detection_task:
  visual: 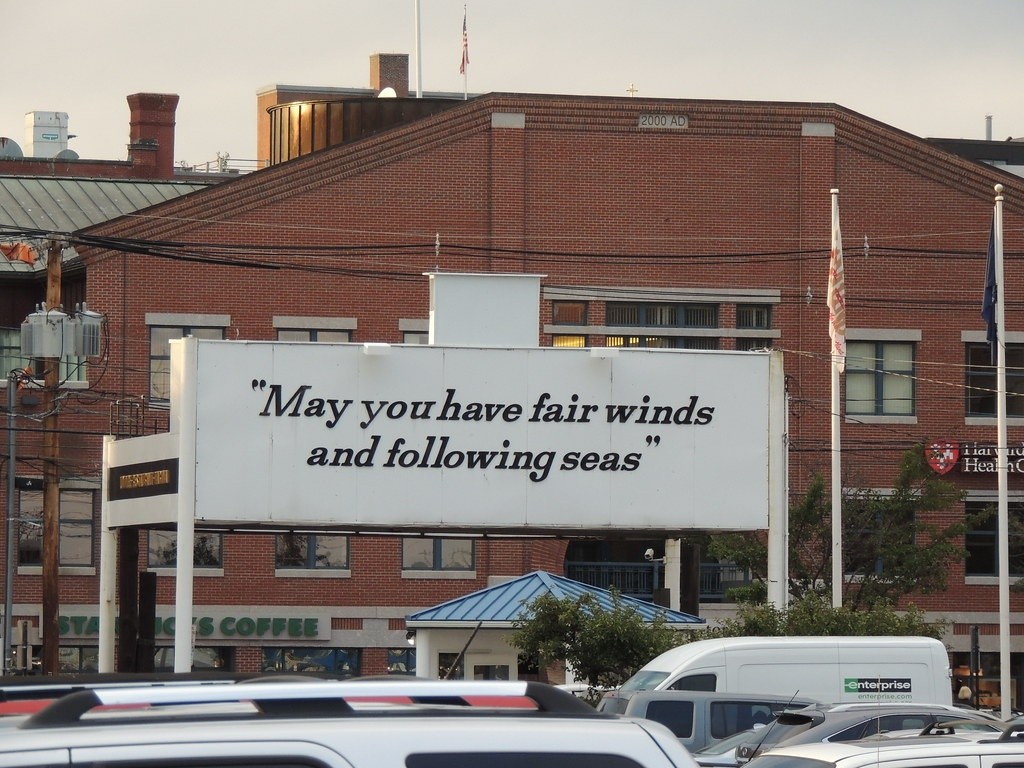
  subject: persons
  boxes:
[957,686,975,708]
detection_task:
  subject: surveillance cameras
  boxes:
[644,547,654,559]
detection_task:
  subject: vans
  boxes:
[594,636,954,752]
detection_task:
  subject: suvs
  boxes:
[689,700,1024,766]
[0,681,699,768]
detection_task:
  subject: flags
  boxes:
[459,16,470,75]
[826,206,847,374]
[980,214,998,345]
